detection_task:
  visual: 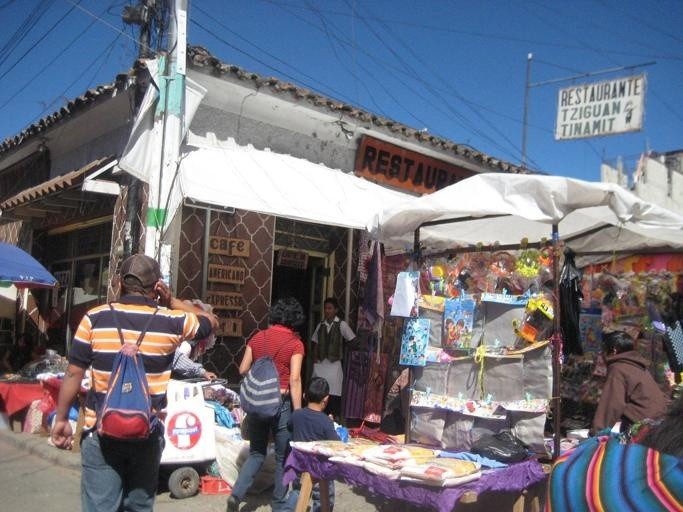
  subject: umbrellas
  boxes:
[0,242,59,289]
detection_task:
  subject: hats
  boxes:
[120,253,164,287]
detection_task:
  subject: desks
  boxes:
[0,371,94,454]
[283,438,550,512]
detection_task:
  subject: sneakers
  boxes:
[226,495,241,512]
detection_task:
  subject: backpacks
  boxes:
[240,330,299,420]
[96,303,160,442]
